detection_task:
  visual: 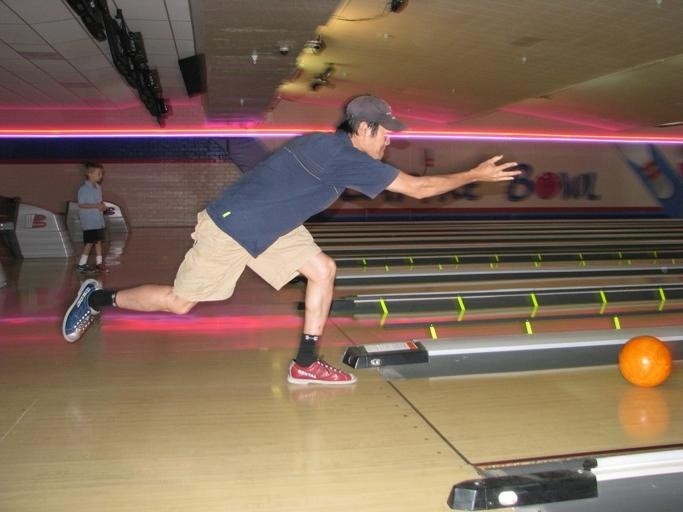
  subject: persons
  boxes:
[62,95,521,384]
[76,160,110,268]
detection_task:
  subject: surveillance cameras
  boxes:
[280,48,289,55]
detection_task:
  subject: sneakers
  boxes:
[75,263,111,276]
[61,278,104,345]
[286,353,359,387]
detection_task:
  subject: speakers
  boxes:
[178,54,202,96]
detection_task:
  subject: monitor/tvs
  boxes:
[138,62,162,126]
[67,0,105,41]
[104,11,138,89]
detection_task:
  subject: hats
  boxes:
[346,93,407,134]
